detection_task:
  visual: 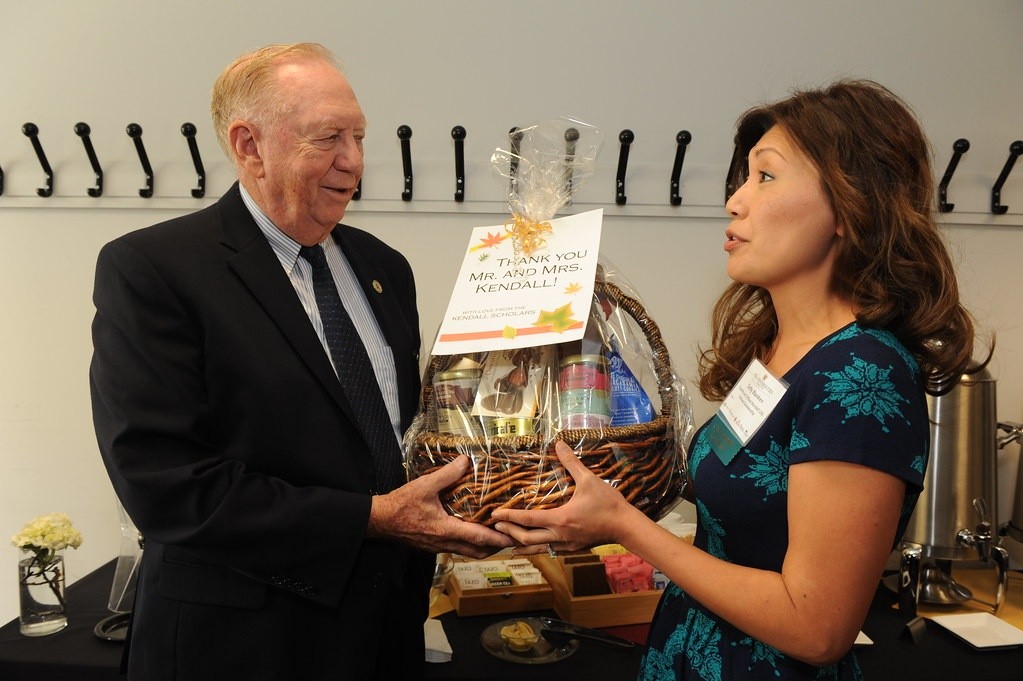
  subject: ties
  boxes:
[300,245,407,496]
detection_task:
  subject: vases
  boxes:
[18,547,67,637]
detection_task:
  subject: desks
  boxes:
[0,544,1023,681]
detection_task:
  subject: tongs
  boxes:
[540,616,627,649]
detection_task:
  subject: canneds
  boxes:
[432,368,487,438]
[488,416,542,437]
[553,353,613,430]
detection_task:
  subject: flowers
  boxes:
[12,513,83,620]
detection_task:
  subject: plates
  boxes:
[480,616,578,663]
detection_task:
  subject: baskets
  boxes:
[410,283,688,555]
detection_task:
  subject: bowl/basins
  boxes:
[428,552,454,609]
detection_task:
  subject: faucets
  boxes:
[955,495,992,564]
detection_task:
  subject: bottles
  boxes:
[551,354,613,437]
[433,367,484,439]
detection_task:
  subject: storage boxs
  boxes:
[471,345,557,423]
[449,548,663,631]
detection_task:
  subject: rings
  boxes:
[546,543,557,559]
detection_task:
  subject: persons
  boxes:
[48,567,61,590]
[491,81,997,681]
[89,40,515,681]
